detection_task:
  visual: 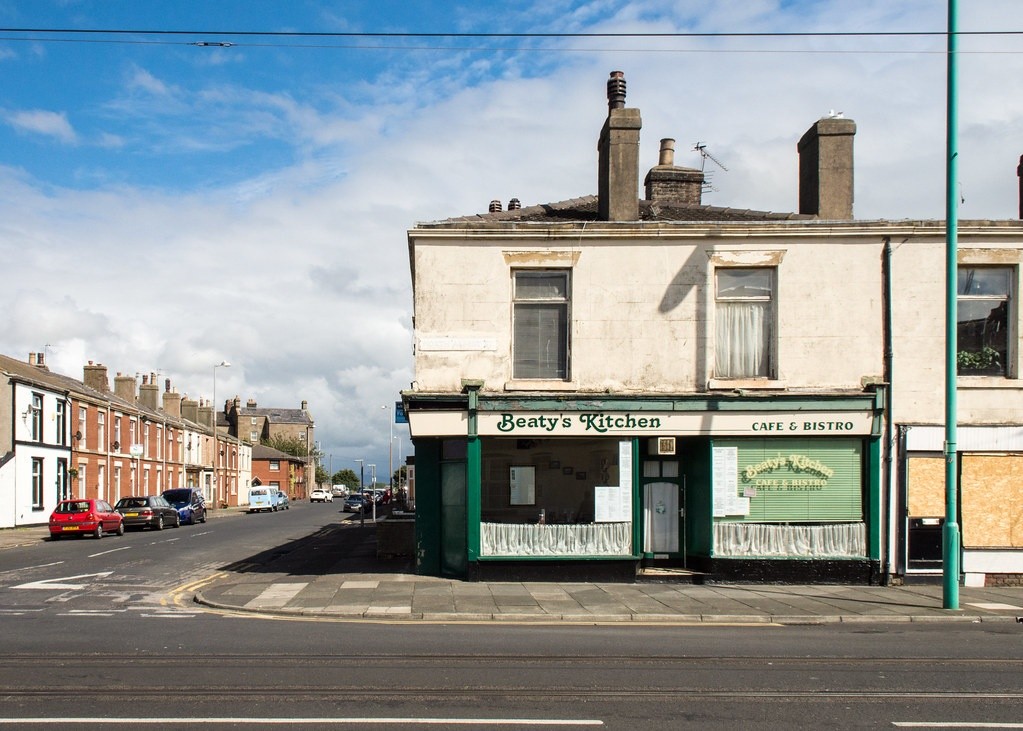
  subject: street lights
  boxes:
[306,425,317,496]
[382,406,394,515]
[316,440,322,467]
[354,459,364,530]
[367,464,376,522]
[213,361,231,512]
[394,436,402,491]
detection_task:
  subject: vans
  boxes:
[248,486,280,512]
[333,485,350,496]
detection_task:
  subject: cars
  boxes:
[332,489,345,497]
[114,495,180,532]
[360,488,386,504]
[277,491,289,510]
[48,499,125,539]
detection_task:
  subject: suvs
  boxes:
[159,487,207,524]
[343,493,373,512]
[310,489,334,503]
